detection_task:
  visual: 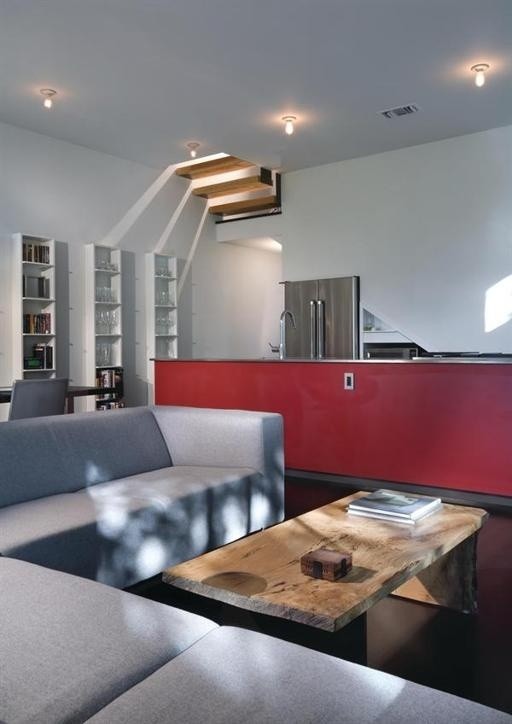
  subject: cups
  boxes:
[96,287,118,302]
[97,343,111,366]
[159,264,169,276]
[155,289,169,305]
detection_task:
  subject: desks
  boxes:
[0,386,108,414]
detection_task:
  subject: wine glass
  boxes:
[155,314,175,335]
[95,311,118,334]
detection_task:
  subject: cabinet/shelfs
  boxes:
[12,233,179,412]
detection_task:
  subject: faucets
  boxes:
[268,310,296,358]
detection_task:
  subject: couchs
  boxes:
[0,555,512,724]
[0,404,285,590]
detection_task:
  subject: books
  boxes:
[349,506,443,525]
[23,244,53,370]
[96,369,124,410]
[349,488,442,518]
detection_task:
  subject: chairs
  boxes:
[8,378,68,421]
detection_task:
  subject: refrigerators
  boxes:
[284,276,359,360]
